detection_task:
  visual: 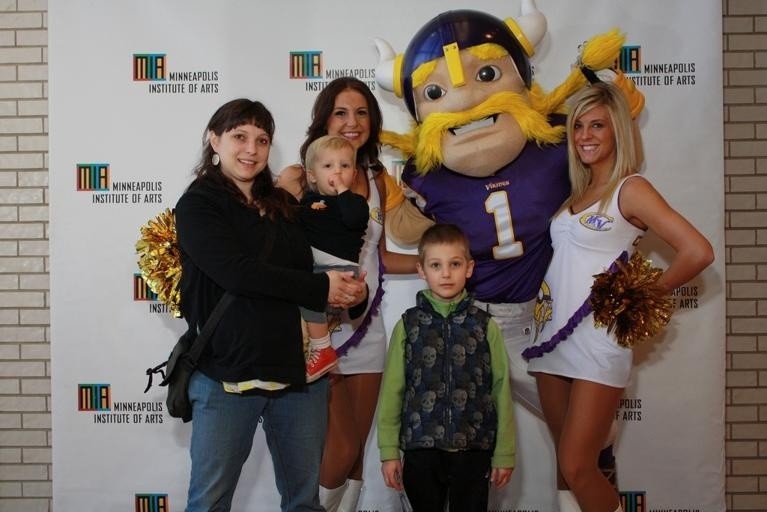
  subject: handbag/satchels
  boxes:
[159,328,200,423]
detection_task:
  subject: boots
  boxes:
[317,477,365,512]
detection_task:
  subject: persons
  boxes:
[364,1,567,512]
[294,135,371,384]
[523,83,712,512]
[161,98,367,511]
[281,77,421,511]
[377,224,516,512]
